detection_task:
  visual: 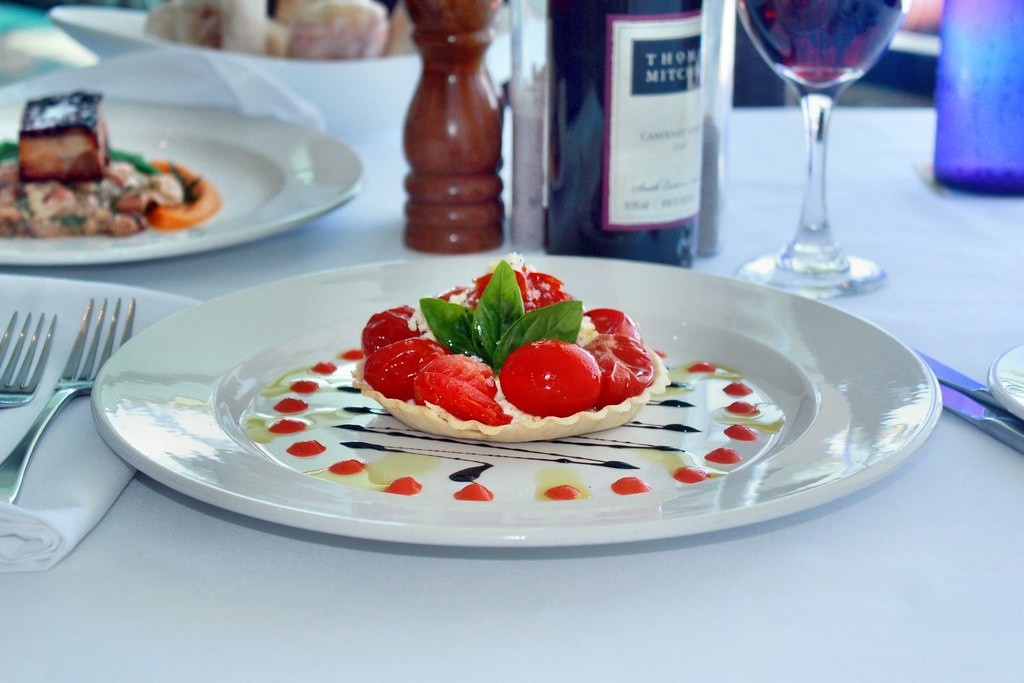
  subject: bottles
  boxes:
[931,0,1024,200]
[539,0,706,270]
[505,0,722,259]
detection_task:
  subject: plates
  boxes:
[986,338,1024,422]
[89,252,942,550]
[0,100,366,268]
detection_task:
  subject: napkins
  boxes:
[2,274,206,575]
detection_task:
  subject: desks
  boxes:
[1,105,1024,683]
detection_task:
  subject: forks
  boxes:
[0,296,140,503]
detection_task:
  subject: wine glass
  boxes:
[726,0,914,303]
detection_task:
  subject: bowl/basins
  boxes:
[48,3,420,127]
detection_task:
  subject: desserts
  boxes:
[144,0,416,58]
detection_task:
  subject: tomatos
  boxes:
[364,274,655,416]
